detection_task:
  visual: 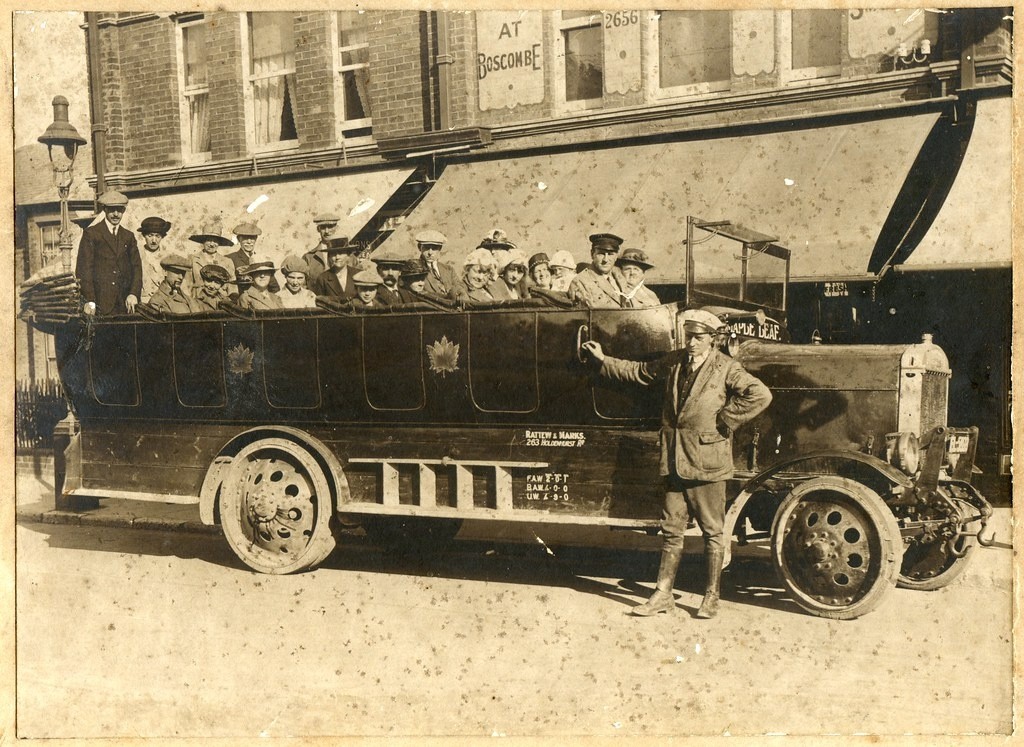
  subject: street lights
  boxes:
[36,94,89,272]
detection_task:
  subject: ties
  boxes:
[607,277,616,292]
[112,227,117,245]
[685,357,694,376]
[432,264,441,280]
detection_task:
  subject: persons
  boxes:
[186,221,237,290]
[528,251,555,293]
[75,191,143,317]
[274,253,317,310]
[567,233,642,309]
[451,226,530,307]
[398,230,474,310]
[226,224,280,294]
[343,251,422,307]
[304,210,359,294]
[314,237,362,305]
[193,265,234,312]
[616,247,662,310]
[149,254,213,314]
[236,252,284,310]
[547,251,591,296]
[582,310,772,620]
[134,216,173,307]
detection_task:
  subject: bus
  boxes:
[28,211,997,623]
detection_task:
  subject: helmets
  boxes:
[401,259,430,276]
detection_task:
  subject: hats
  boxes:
[160,254,194,271]
[233,222,261,236]
[462,229,577,279]
[683,309,722,333]
[200,265,231,284]
[97,191,128,206]
[188,224,234,246]
[371,252,406,265]
[281,256,310,276]
[313,213,340,223]
[136,217,171,236]
[416,230,445,245]
[352,270,384,286]
[615,248,654,270]
[589,234,623,250]
[239,255,279,276]
[318,237,359,251]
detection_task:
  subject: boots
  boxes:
[632,545,683,615]
[697,547,724,618]
